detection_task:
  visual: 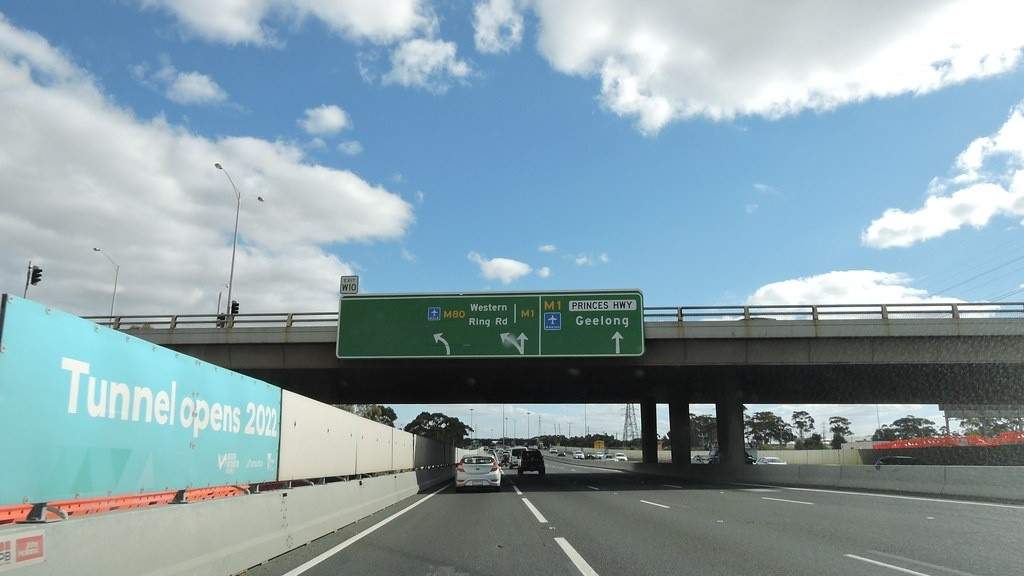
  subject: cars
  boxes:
[692,448,720,466]
[745,451,756,465]
[455,454,503,492]
[549,448,557,454]
[558,450,565,457]
[484,446,512,467]
[874,456,927,465]
[573,450,627,462]
[756,456,787,465]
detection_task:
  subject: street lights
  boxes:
[470,409,474,440]
[214,163,266,328]
[93,247,119,327]
[505,417,508,437]
[215,283,229,328]
[527,412,530,440]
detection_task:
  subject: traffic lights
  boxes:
[30,266,43,286]
[231,300,239,314]
[217,313,225,328]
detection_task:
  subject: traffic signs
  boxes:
[336,291,541,359]
[540,290,645,357]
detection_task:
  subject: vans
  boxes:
[509,447,527,469]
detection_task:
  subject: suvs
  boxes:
[517,451,545,476]
